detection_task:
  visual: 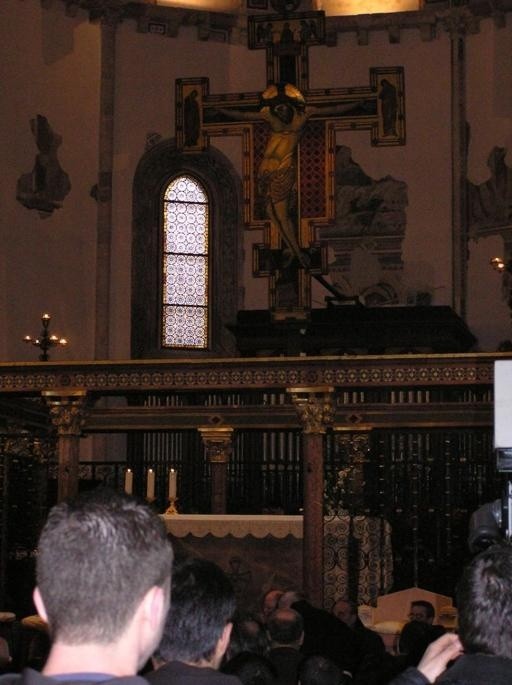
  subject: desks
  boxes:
[155,513,397,607]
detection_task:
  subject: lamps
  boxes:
[22,313,69,360]
[488,254,512,275]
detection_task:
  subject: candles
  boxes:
[168,468,177,500]
[125,468,133,496]
[146,469,156,496]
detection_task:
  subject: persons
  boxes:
[256,20,317,43]
[218,81,360,270]
[378,79,398,136]
[184,90,200,146]
[2,486,510,684]
